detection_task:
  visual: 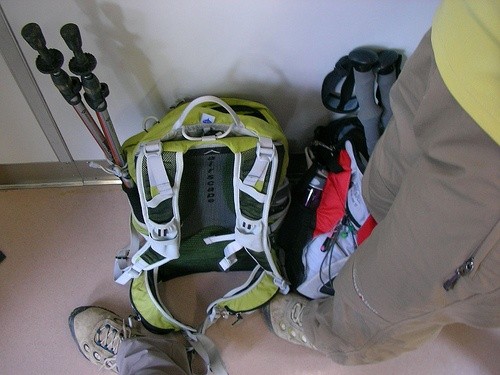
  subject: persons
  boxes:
[69,306,196,375]
[256,0,500,367]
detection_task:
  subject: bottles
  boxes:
[304,176,326,212]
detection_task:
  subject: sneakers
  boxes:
[68,305,147,374]
[259,290,318,350]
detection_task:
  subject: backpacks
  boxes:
[114,91,290,375]
